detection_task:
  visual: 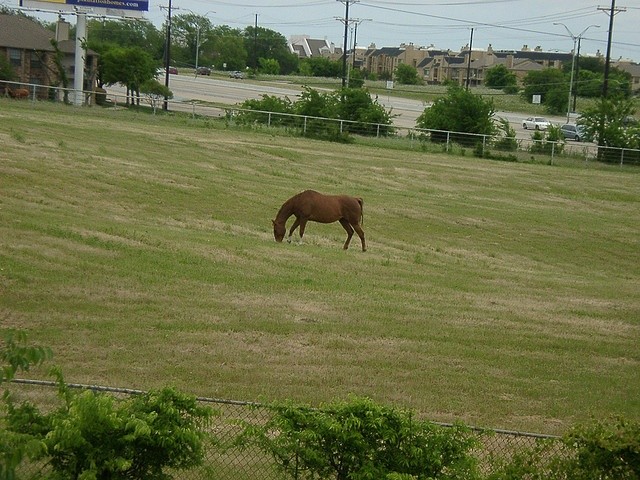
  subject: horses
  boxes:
[271,189,367,252]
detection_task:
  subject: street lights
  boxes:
[182,8,216,69]
[553,22,601,123]
[335,18,373,89]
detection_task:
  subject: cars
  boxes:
[559,124,593,142]
[194,67,211,76]
[228,71,243,79]
[166,66,178,75]
[522,116,553,131]
